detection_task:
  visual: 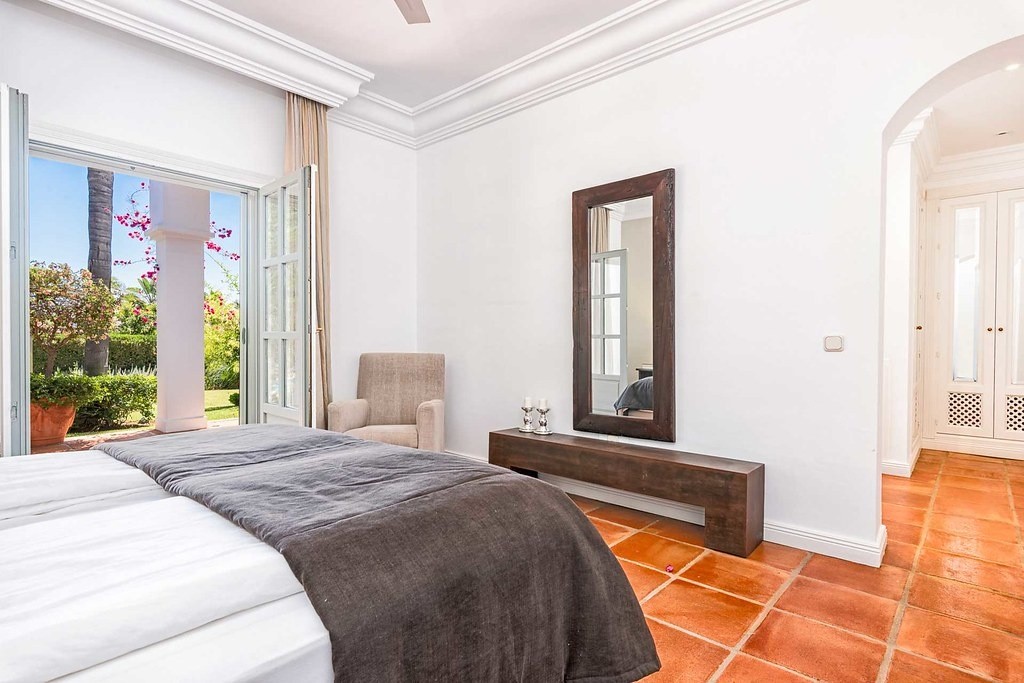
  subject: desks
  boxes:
[488,427,766,557]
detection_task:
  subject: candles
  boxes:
[539,398,548,408]
[524,397,533,406]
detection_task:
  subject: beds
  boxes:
[0,422,659,683]
[614,376,654,419]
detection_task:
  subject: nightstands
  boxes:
[635,367,653,378]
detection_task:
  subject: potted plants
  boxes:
[30,369,95,447]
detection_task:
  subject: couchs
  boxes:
[327,351,445,453]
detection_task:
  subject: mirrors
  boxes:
[572,169,674,442]
